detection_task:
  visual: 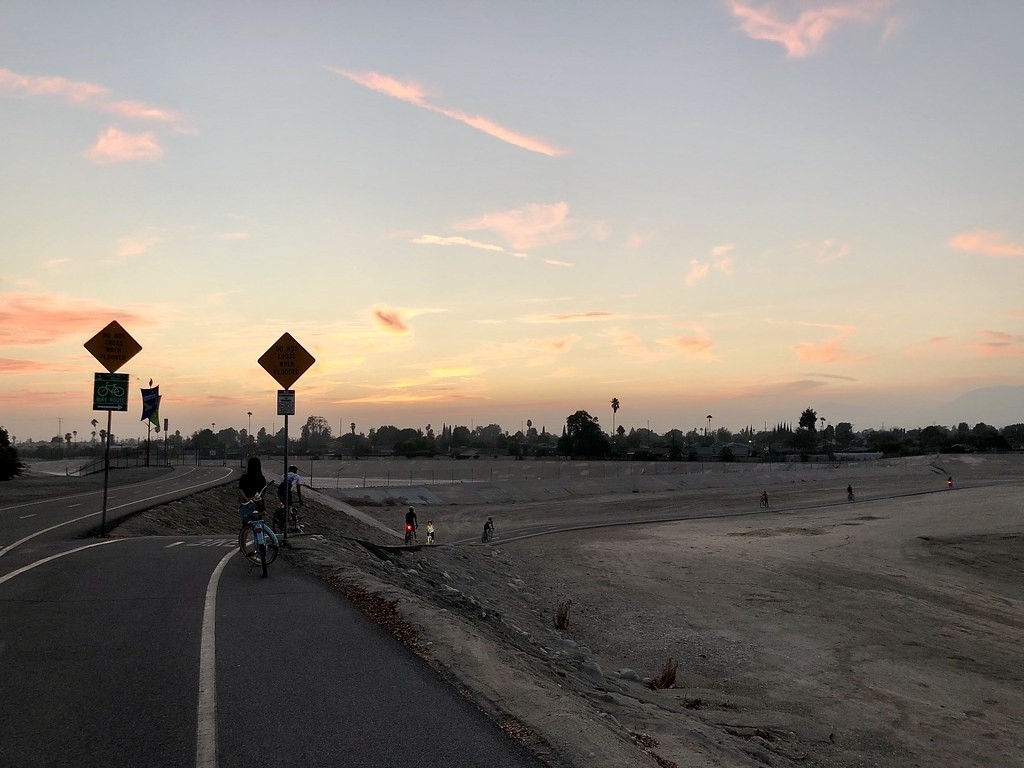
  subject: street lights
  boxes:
[248,412,252,435]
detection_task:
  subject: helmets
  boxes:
[409,506,414,511]
[288,465,298,471]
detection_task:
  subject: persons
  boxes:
[277,465,304,521]
[426,521,435,542]
[484,517,494,538]
[847,484,852,501]
[947,477,953,487]
[238,457,267,553]
[761,491,768,507]
[405,506,419,545]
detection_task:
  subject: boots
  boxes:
[288,505,293,521]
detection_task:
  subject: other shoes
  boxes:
[415,539,418,543]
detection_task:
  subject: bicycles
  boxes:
[273,500,300,534]
[482,528,494,542]
[408,525,417,545]
[760,496,768,508]
[427,528,434,545]
[848,493,855,501]
[238,479,280,578]
